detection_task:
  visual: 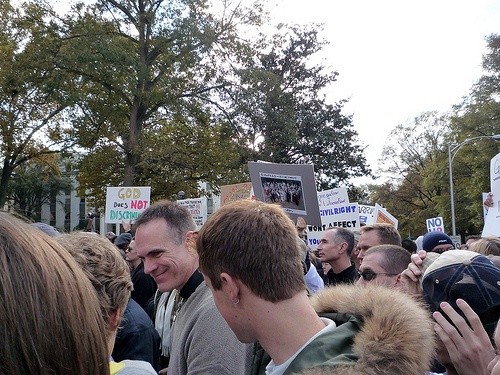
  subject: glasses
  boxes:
[128,247,132,252]
[432,247,453,254]
[357,268,393,281]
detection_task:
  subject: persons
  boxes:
[54,231,158,375]
[264,181,301,205]
[0,212,111,375]
[132,202,255,375]
[195,200,436,375]
[17,192,500,375]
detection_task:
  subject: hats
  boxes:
[415,249,500,326]
[423,231,455,252]
[115,233,132,249]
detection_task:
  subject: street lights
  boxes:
[448,135,500,237]
